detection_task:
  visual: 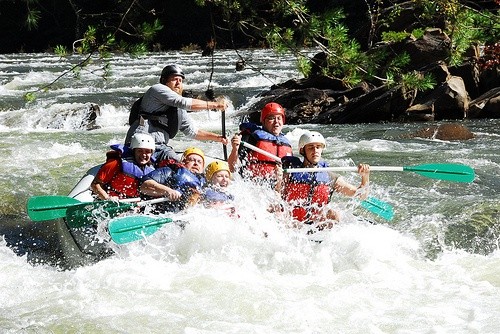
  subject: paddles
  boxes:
[221,97,231,163]
[66,196,175,228]
[25,195,88,223]
[235,136,395,221]
[108,205,209,247]
[282,162,478,184]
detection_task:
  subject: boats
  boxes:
[53,161,389,269]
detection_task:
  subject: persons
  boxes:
[124,65,228,153]
[91,103,368,243]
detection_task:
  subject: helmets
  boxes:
[260,103,286,124]
[298,131,327,155]
[130,134,156,151]
[181,147,204,174]
[161,64,186,80]
[205,161,231,183]
